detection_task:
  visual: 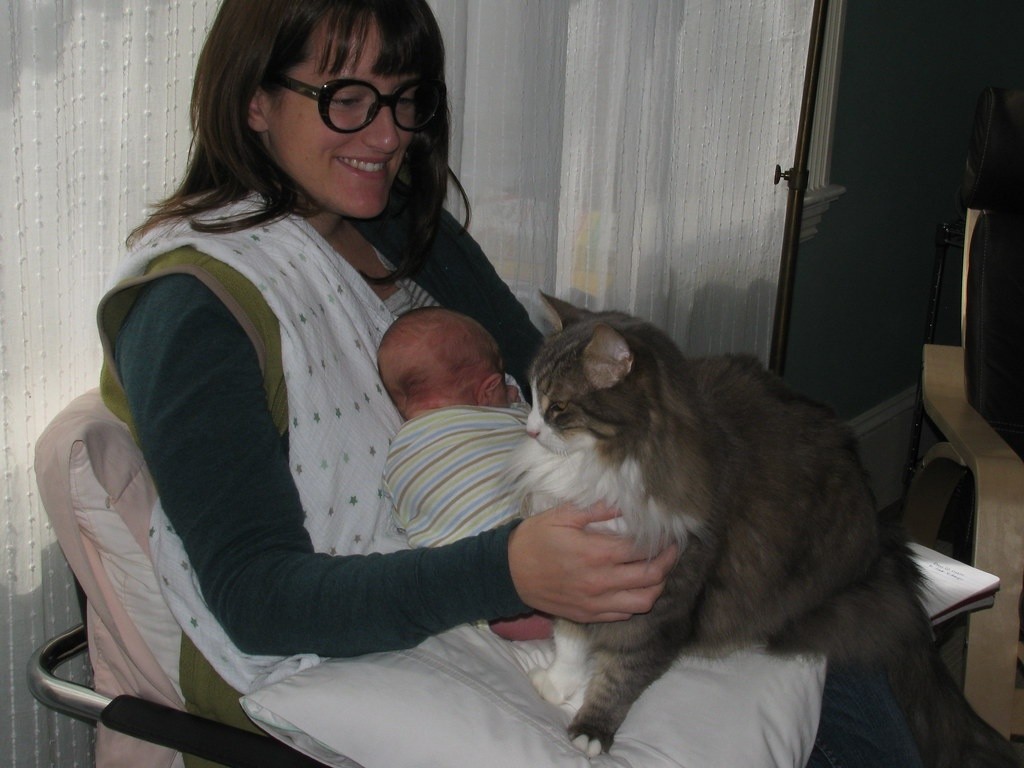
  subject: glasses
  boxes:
[269,67,444,131]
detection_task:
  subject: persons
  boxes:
[96,1,920,767]
[378,304,555,643]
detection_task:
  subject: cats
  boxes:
[521,289,954,768]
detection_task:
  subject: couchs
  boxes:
[30,277,977,765]
